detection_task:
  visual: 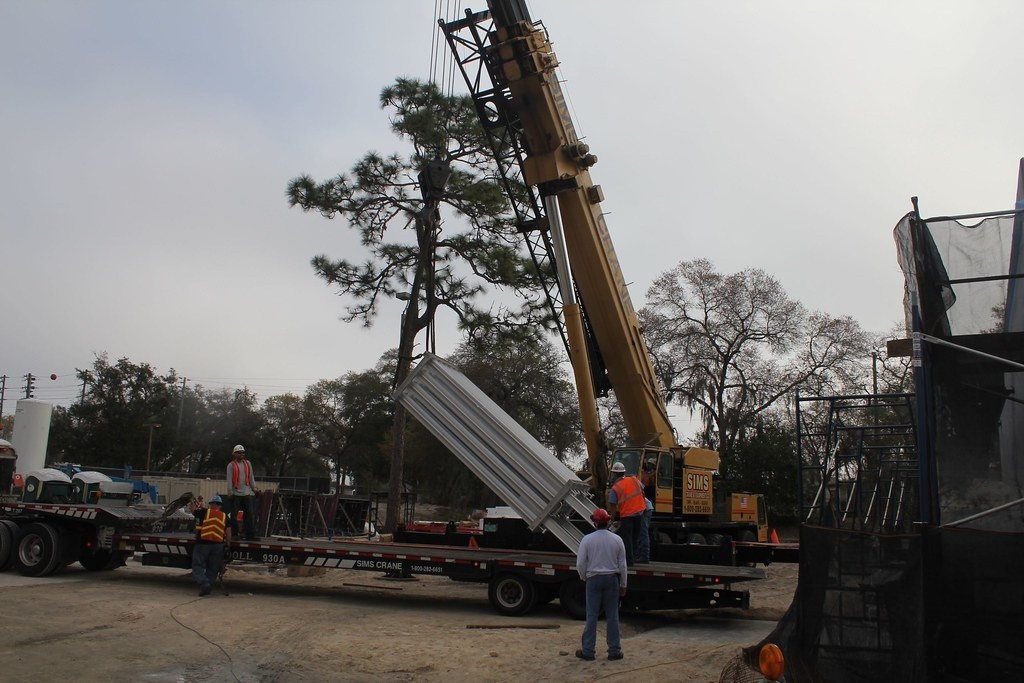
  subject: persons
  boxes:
[191,496,206,518]
[608,462,648,568]
[192,496,231,597]
[226,445,261,540]
[576,509,627,660]
[635,498,654,563]
[642,459,656,490]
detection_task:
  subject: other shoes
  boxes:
[575,649,596,660]
[635,558,649,564]
[199,586,212,597]
[609,651,624,660]
[246,535,261,541]
[626,562,633,568]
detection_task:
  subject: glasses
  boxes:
[236,451,245,454]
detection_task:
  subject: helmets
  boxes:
[209,495,222,503]
[590,509,611,522]
[198,496,203,499]
[610,462,626,472]
[233,444,245,453]
[648,458,656,465]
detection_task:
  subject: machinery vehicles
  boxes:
[378,0,770,623]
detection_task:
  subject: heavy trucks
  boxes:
[0,438,770,619]
